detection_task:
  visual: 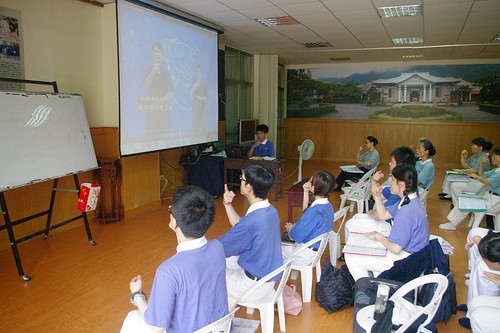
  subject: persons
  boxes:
[216,163,284,311]
[437,135,493,200]
[457,228,500,333]
[144,42,175,102]
[439,146,500,231]
[280,170,334,266]
[189,65,208,130]
[248,124,276,157]
[119,184,234,333]
[335,136,379,192]
[343,137,436,281]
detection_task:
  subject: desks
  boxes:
[223,157,287,203]
[285,177,329,242]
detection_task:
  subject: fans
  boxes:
[292,139,315,186]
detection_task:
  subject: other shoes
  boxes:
[333,186,342,192]
[457,304,468,313]
[439,222,456,230]
[438,193,452,201]
[460,318,471,330]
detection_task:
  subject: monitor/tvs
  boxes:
[237,118,257,146]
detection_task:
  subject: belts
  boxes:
[489,191,500,196]
[308,246,318,251]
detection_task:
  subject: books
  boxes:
[446,170,469,182]
[338,164,364,173]
[455,192,487,212]
[342,232,388,257]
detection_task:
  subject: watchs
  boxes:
[129,291,147,306]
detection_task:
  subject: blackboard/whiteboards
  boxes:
[0,91,101,192]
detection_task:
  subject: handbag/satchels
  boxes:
[275,283,302,316]
[318,262,356,314]
[77,182,102,212]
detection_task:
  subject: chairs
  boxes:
[184,167,500,333]
